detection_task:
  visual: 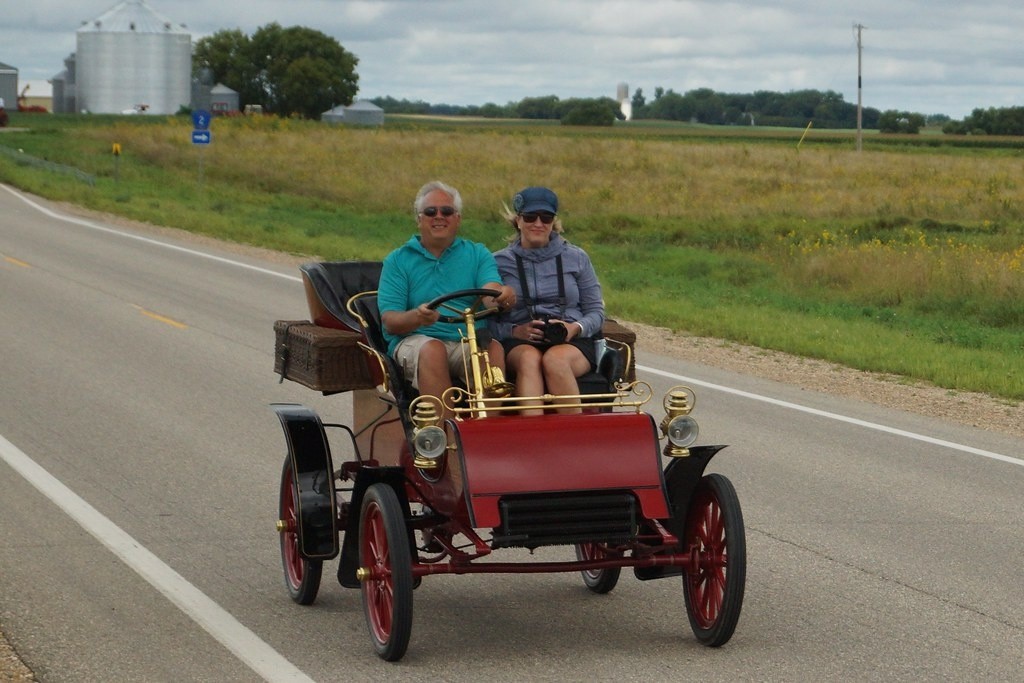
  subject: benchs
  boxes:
[296,263,621,396]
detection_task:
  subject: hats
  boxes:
[512,187,558,216]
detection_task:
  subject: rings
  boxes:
[507,303,509,306]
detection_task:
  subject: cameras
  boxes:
[533,313,568,343]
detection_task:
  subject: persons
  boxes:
[377,180,517,428]
[491,187,605,416]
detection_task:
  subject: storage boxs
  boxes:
[601,316,636,382]
[273,320,369,390]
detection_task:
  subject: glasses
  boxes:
[418,206,458,217]
[517,212,556,224]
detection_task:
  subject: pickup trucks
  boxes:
[208,102,241,116]
